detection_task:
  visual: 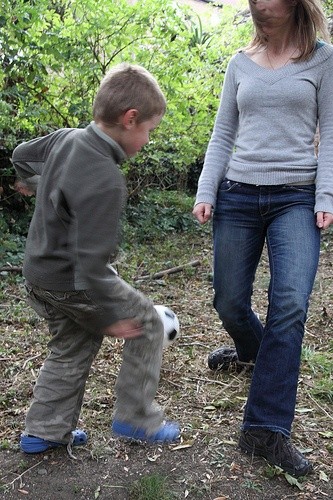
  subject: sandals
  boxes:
[19,430,87,453]
[110,416,179,444]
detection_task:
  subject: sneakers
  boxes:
[207,348,238,372]
[238,425,311,478]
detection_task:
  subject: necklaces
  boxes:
[264,44,299,69]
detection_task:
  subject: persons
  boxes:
[10,64,182,455]
[193,0,333,478]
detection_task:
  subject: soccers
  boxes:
[153,305,180,347]
